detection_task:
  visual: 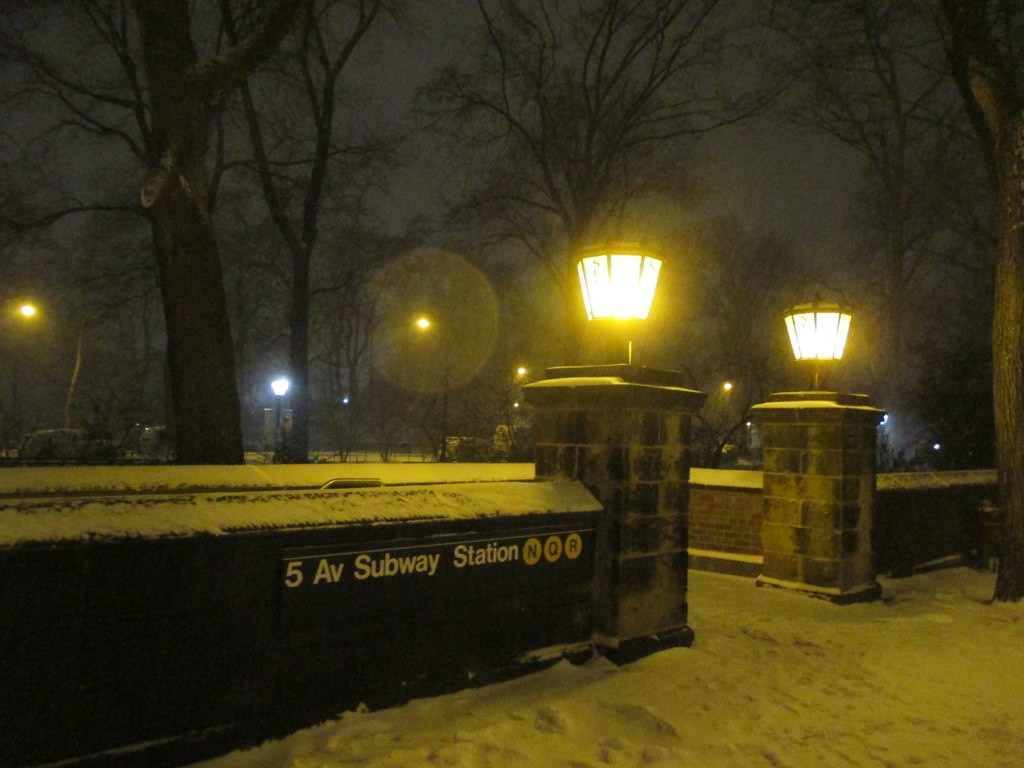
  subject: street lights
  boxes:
[270,378,289,462]
